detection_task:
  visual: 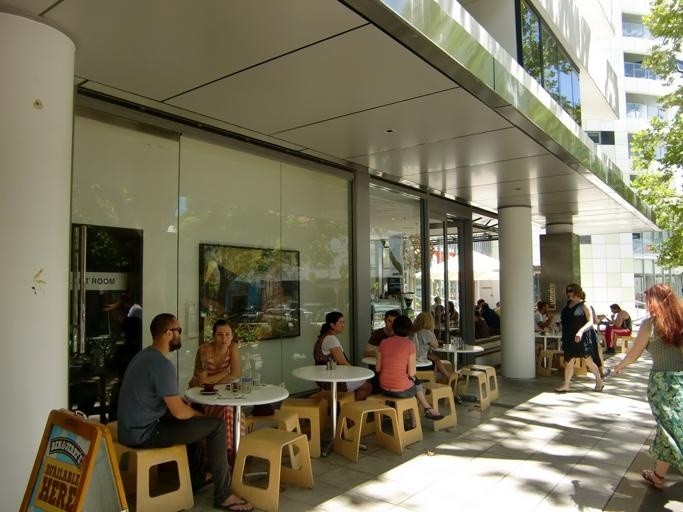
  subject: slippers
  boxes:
[213,492,254,512]
[194,472,213,490]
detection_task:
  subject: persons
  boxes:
[533,301,555,333]
[430,292,501,379]
[114,292,142,380]
[603,305,632,354]
[188,319,246,473]
[554,284,604,392]
[614,283,683,489]
[375,315,444,429]
[313,312,373,401]
[363,310,401,392]
[408,311,463,382]
[117,313,254,512]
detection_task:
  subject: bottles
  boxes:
[241,352,253,392]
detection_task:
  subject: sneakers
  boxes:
[604,347,615,354]
[555,385,570,392]
[592,384,605,392]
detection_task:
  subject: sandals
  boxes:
[423,407,445,421]
[641,470,666,489]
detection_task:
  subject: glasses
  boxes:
[566,289,575,293]
[166,328,182,335]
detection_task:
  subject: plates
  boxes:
[198,389,218,395]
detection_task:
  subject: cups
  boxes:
[230,377,241,394]
[544,327,549,335]
[253,372,261,386]
[555,326,559,335]
[327,357,336,370]
[203,382,214,390]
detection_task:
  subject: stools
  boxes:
[614,335,635,353]
[537,349,567,377]
